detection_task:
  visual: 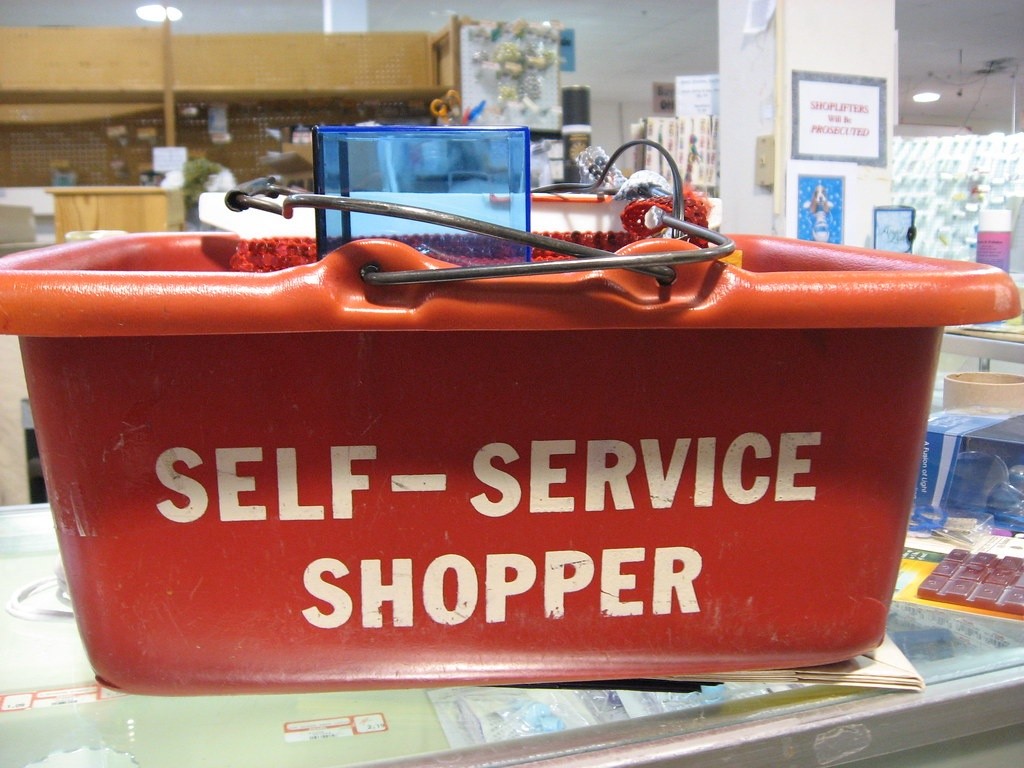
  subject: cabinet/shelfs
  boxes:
[1,14,463,191]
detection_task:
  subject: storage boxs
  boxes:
[1,215,1024,700]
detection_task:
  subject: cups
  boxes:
[941,373,1024,416]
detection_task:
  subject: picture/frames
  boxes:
[788,68,890,168]
